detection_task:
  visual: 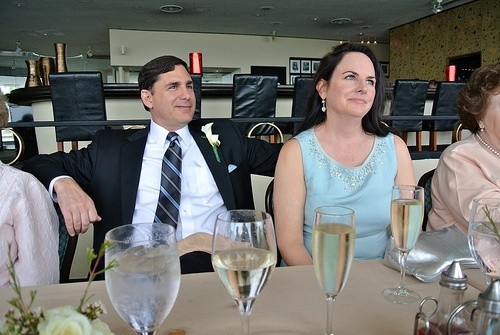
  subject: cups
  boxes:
[40,57,54,86]
[468,198,500,285]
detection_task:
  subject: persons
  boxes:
[23,56,285,281]
[0,87,60,288]
[425,62,500,273]
[273,43,415,267]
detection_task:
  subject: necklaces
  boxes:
[476,133,500,157]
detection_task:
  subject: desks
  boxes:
[0,260,483,335]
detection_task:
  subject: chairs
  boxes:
[423,82,468,152]
[48,72,124,152]
[277,77,317,145]
[382,81,430,153]
[232,75,279,144]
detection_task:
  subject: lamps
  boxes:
[431,0,443,14]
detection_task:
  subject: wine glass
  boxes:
[381,185,425,305]
[312,206,355,335]
[106,223,181,334]
[211,209,278,335]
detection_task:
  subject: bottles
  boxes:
[25,60,41,87]
[472,279,500,335]
[418,262,474,335]
[54,43,68,72]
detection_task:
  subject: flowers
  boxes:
[0,238,120,335]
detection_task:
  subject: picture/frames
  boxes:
[379,61,390,79]
[289,57,324,85]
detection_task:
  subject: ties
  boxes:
[151,133,183,248]
